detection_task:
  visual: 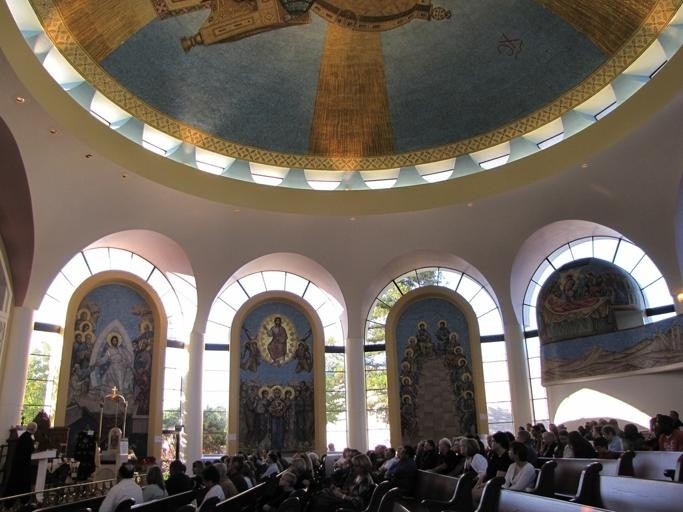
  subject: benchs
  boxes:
[83,445,683,511]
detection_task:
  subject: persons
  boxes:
[10,422,38,506]
[542,271,623,336]
[240,314,314,450]
[68,308,154,413]
[642,411,682,451]
[397,320,478,437]
[415,418,644,491]
[316,439,416,512]
[98,451,319,512]
